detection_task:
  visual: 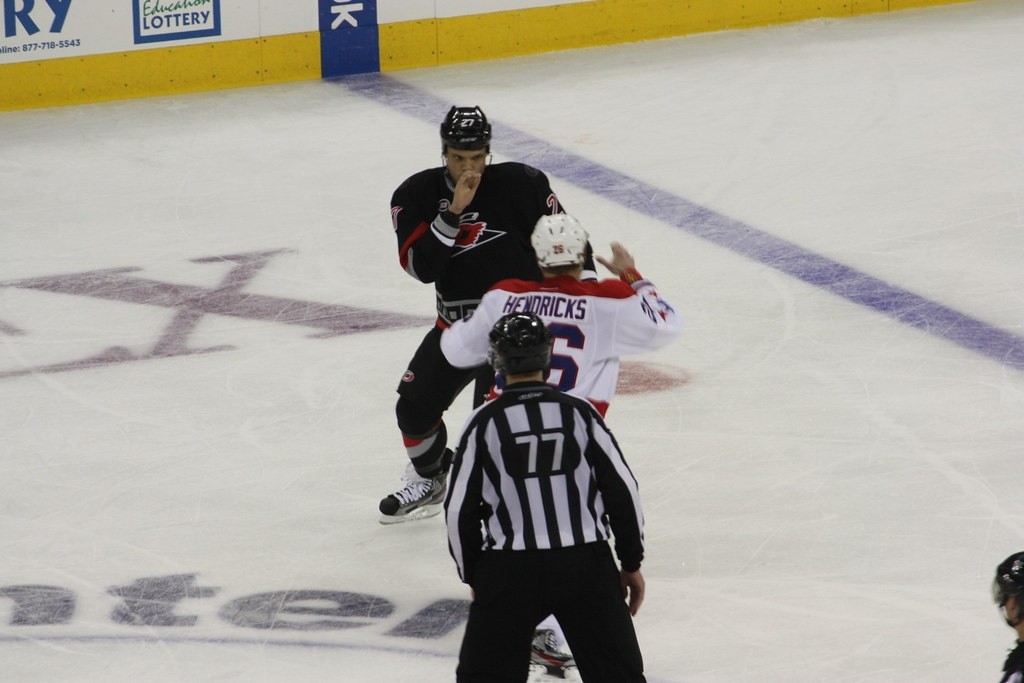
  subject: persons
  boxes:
[441,212,681,669]
[377,103,595,526]
[991,552,1024,683]
[444,314,648,683]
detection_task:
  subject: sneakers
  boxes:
[378,447,455,524]
[530,626,576,679]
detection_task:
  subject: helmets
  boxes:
[531,211,589,267]
[996,551,1024,593]
[439,105,493,150]
[488,311,551,375]
